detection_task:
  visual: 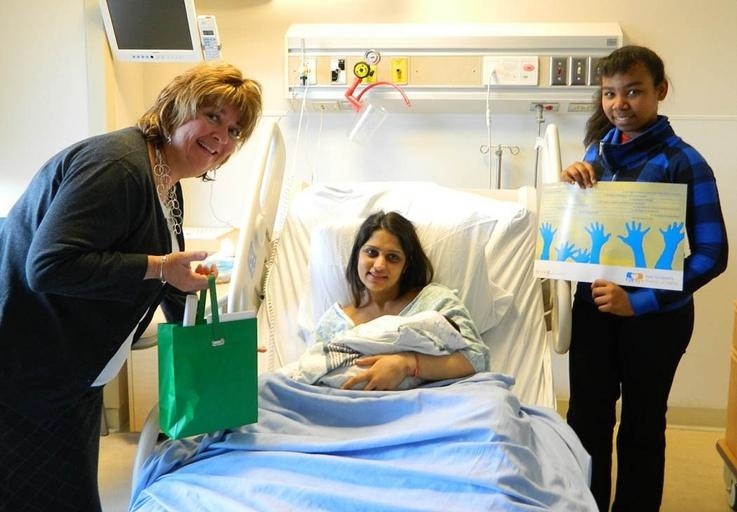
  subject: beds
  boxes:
[124,121,602,512]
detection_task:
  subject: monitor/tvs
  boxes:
[99,0,203,64]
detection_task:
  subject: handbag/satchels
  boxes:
[156,316,258,439]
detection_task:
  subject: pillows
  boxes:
[304,210,509,352]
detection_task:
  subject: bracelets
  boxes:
[159,256,166,283]
[410,352,421,379]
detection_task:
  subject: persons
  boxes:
[0,61,258,512]
[312,212,491,391]
[567,47,727,511]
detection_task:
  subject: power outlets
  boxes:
[528,100,543,114]
[311,100,330,114]
